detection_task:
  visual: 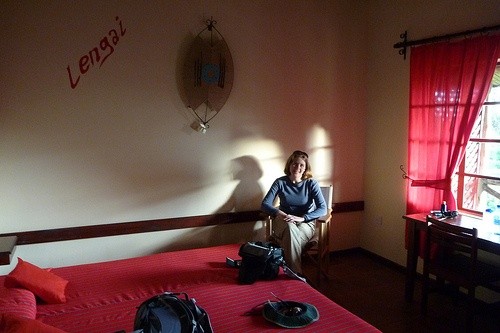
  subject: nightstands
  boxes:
[0,236,17,266]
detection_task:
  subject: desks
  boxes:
[402,208,500,314]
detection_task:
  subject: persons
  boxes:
[261,150,328,277]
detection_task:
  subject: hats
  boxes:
[261,297,320,330]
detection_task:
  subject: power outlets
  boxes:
[374,215,382,225]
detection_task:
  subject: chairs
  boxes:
[420,214,500,333]
[267,183,334,286]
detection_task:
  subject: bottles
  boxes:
[441,202,447,213]
[483,204,500,237]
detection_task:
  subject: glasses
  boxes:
[294,150,309,159]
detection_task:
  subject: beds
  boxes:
[0,238,382,333]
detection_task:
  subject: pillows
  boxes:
[7,257,70,303]
[1,288,36,320]
[1,311,63,333]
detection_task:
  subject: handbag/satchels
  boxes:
[132,291,214,333]
[225,241,308,285]
